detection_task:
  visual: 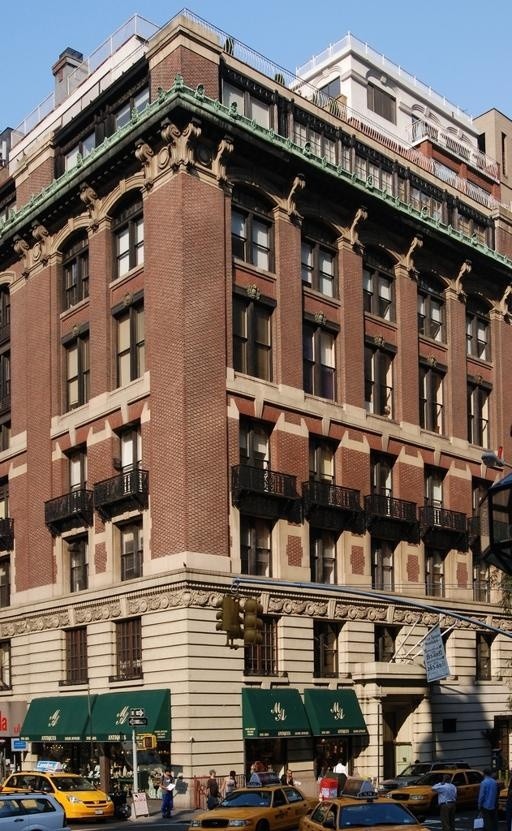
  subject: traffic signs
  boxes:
[128,708,146,718]
[128,717,148,728]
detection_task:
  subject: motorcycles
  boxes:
[108,783,131,818]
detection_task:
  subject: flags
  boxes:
[420,625,450,683]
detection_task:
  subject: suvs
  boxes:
[377,760,471,800]
[0,790,72,830]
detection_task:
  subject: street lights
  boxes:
[481,449,512,471]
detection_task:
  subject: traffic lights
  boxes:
[215,597,239,636]
[142,735,157,749]
[244,598,263,646]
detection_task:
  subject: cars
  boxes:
[498,788,509,812]
[383,769,504,815]
[186,771,317,830]
[0,770,115,824]
[300,776,432,830]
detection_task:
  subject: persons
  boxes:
[219,771,240,797]
[159,767,175,819]
[205,769,224,810]
[333,759,349,778]
[319,760,333,784]
[478,768,498,831]
[432,775,457,831]
[280,770,295,786]
